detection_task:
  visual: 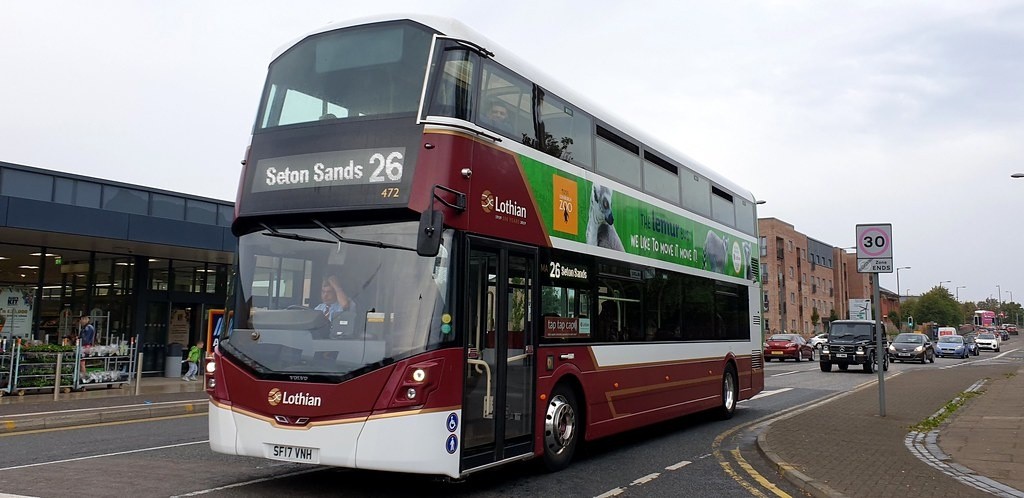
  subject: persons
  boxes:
[75,316,95,373]
[313,274,357,321]
[181,340,204,382]
[480,102,514,135]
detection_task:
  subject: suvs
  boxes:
[820,320,891,376]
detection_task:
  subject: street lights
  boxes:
[940,280,966,304]
[896,267,912,327]
[996,283,1012,304]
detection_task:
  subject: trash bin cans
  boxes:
[165,343,183,377]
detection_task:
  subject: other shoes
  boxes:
[182,376,189,381]
[190,375,198,381]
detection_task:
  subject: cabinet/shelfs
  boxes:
[0,339,15,397]
[77,338,134,392]
[40,314,60,345]
[107,334,139,379]
[13,337,80,395]
[64,308,111,346]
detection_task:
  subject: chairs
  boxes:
[625,306,650,341]
[681,309,727,340]
[597,301,618,342]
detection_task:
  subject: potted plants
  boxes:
[16,343,76,388]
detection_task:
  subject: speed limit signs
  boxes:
[855,223,893,274]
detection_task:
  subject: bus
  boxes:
[203,17,767,476]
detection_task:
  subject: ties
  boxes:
[324,304,331,319]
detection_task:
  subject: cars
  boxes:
[996,322,1017,340]
[974,334,1001,351]
[764,333,814,362]
[808,333,828,349]
[892,333,936,364]
[936,335,980,358]
